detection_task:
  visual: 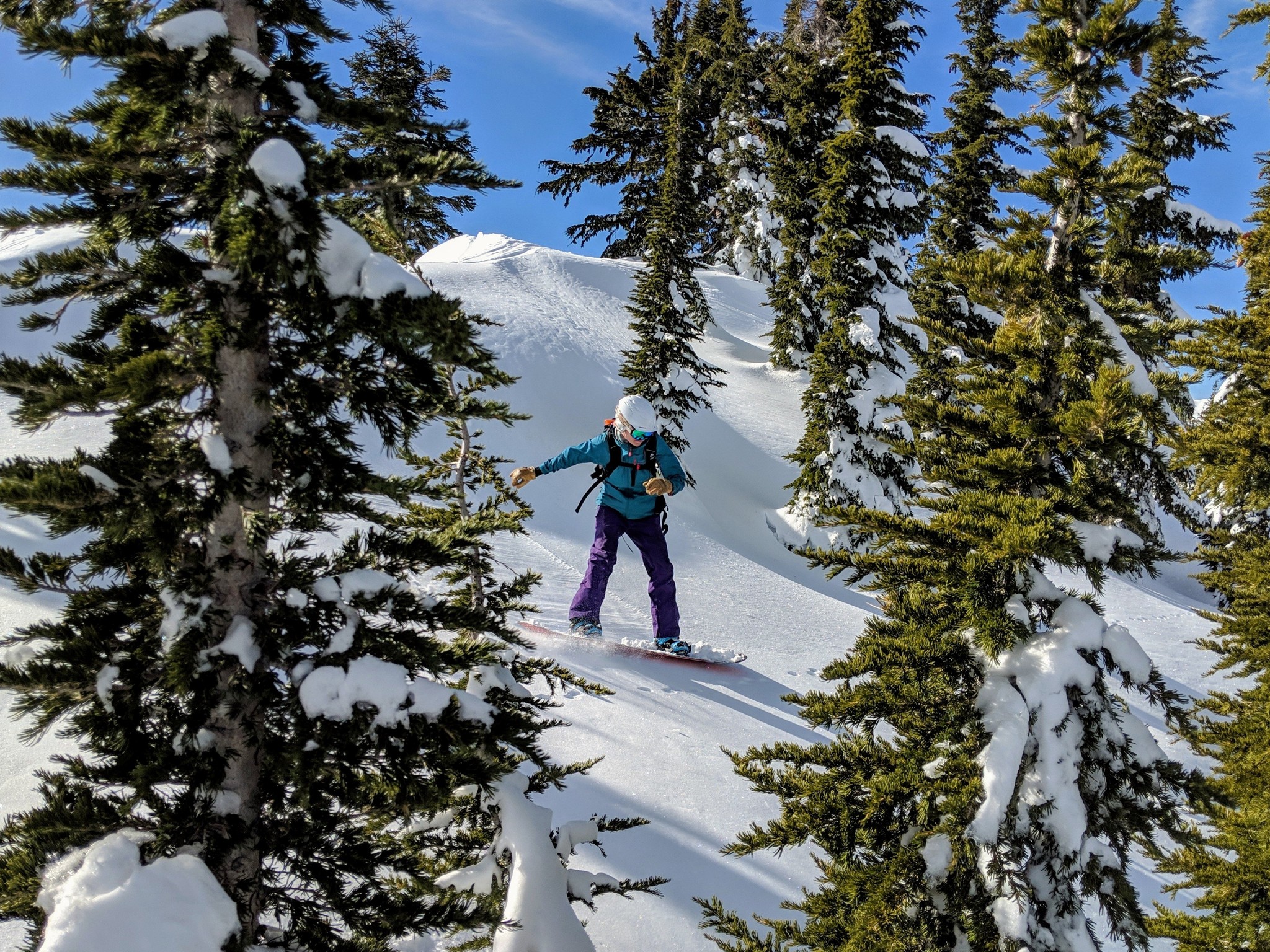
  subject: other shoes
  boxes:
[568,618,602,636]
[653,637,691,656]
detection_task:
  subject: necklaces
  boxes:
[509,395,693,655]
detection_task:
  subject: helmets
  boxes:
[615,394,658,433]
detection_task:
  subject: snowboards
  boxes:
[518,620,747,665]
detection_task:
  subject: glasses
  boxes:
[630,429,654,441]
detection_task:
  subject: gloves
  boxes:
[509,466,536,489]
[643,478,672,496]
